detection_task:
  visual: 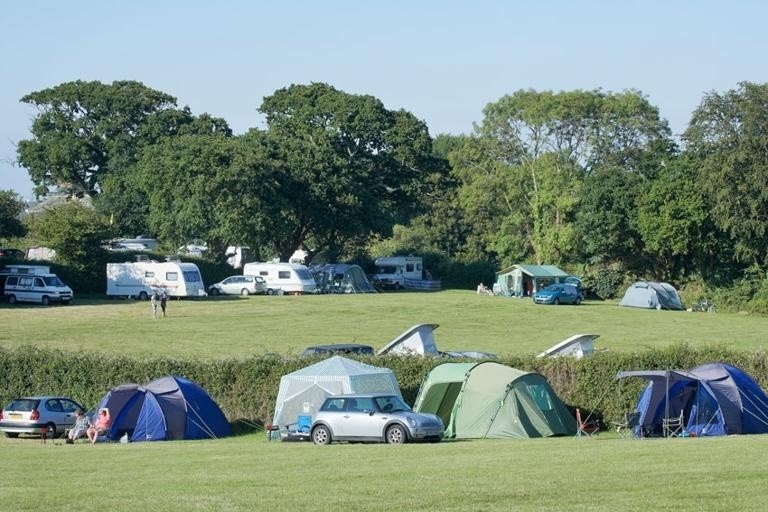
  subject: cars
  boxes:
[0,395,88,439]
[533,277,584,305]
[309,392,444,444]
[437,350,497,359]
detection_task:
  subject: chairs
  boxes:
[610,411,642,439]
[575,408,602,440]
[65,426,90,444]
[282,415,313,442]
[661,408,686,439]
[92,427,112,444]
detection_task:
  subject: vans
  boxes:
[208,275,266,296]
[299,343,373,357]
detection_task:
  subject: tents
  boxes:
[618,280,685,310]
[91,376,235,442]
[409,359,583,440]
[268,354,402,440]
[616,364,768,438]
[312,262,378,294]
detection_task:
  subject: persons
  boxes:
[86,407,111,446]
[160,293,167,317]
[480,282,493,296]
[66,409,88,444]
[151,293,157,319]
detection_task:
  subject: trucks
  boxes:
[373,255,423,290]
[4,273,74,306]
